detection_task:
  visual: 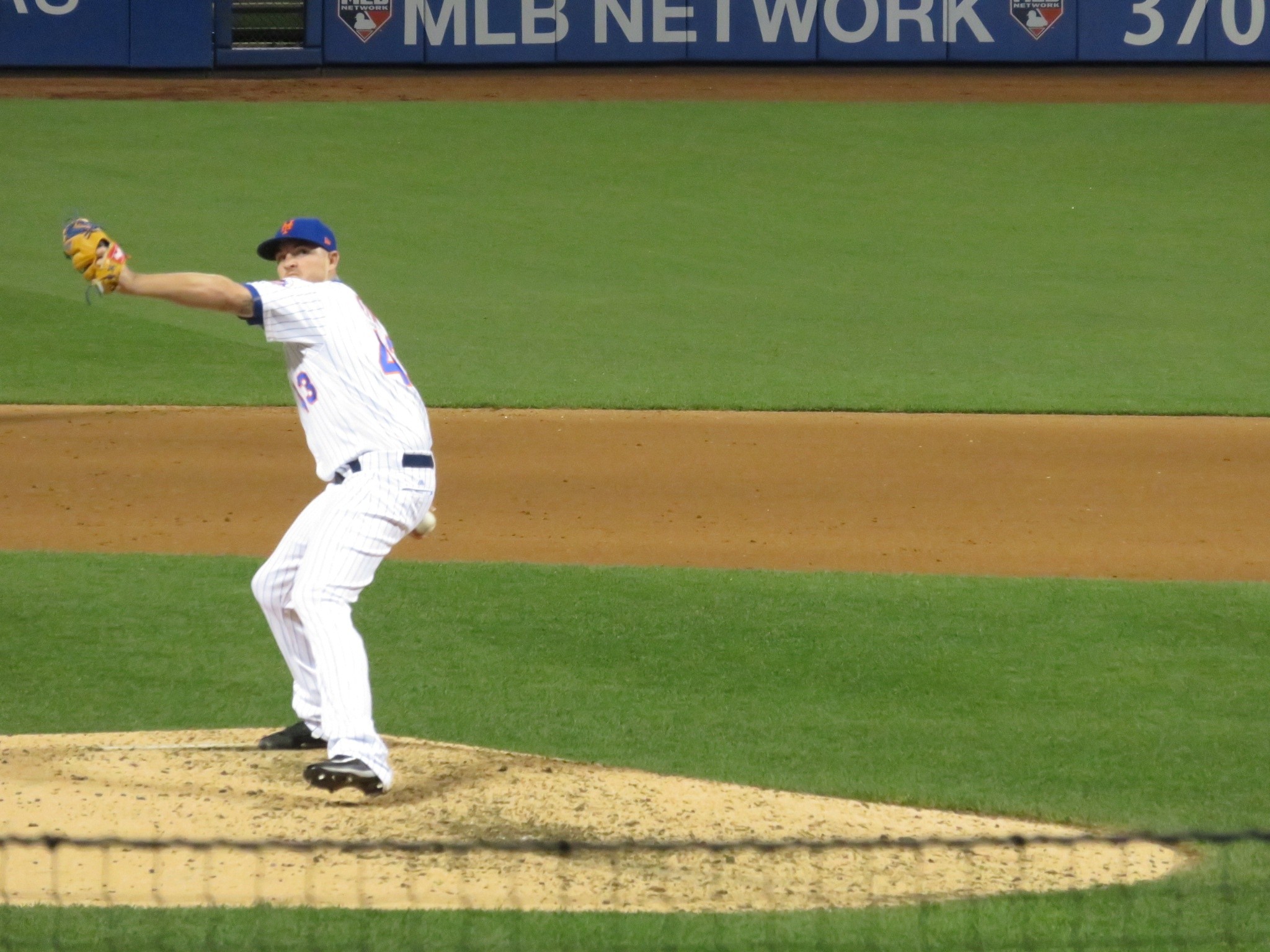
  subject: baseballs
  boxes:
[415,511,436,534]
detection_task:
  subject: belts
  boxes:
[334,453,435,484]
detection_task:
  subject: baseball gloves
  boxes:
[62,217,125,297]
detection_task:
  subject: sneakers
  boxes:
[304,754,386,793]
[256,719,329,750]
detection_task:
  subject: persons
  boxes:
[65,217,439,795]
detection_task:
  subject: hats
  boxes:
[257,218,335,260]
